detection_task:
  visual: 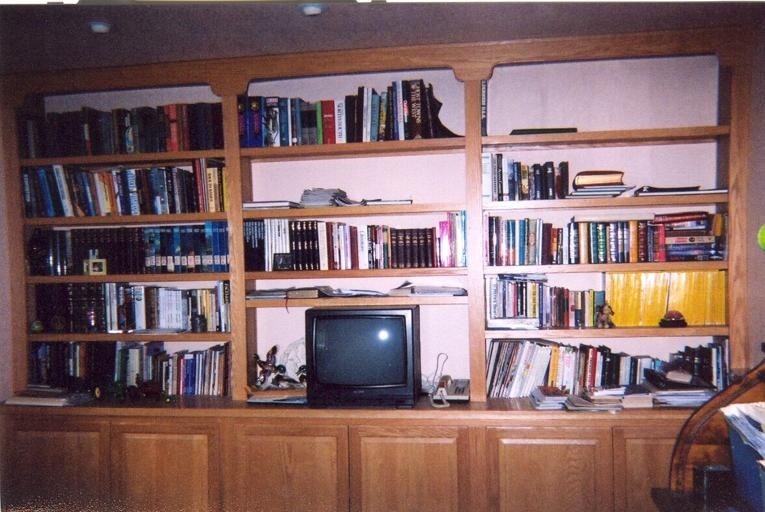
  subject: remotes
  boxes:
[246,395,306,405]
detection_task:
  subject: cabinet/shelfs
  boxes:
[0,409,221,512]
[220,410,481,512]
[2,28,765,399]
[482,409,736,512]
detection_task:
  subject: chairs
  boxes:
[670,360,765,512]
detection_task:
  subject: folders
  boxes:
[644,368,717,389]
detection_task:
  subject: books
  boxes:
[480,79,487,136]
[482,270,727,330]
[242,209,468,274]
[483,338,730,411]
[29,280,233,337]
[21,157,226,220]
[236,78,465,148]
[244,281,471,298]
[239,187,414,210]
[4,342,230,409]
[17,101,226,159]
[23,223,232,278]
[509,127,577,135]
[481,152,729,202]
[483,209,728,267]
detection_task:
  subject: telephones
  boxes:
[428,375,470,408]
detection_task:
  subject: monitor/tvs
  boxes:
[306,307,420,408]
